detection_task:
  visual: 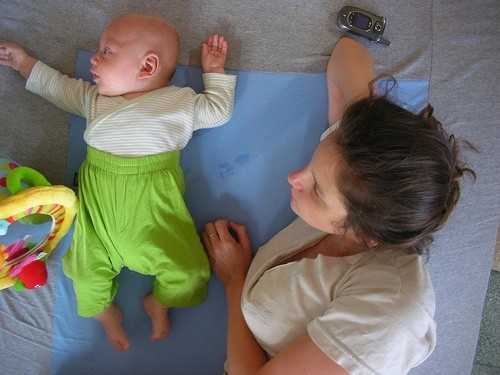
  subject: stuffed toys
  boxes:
[1,153,80,295]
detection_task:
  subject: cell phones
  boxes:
[337,6,390,45]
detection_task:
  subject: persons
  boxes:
[1,12,241,356]
[197,35,485,375]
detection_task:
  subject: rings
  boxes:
[208,232,218,240]
